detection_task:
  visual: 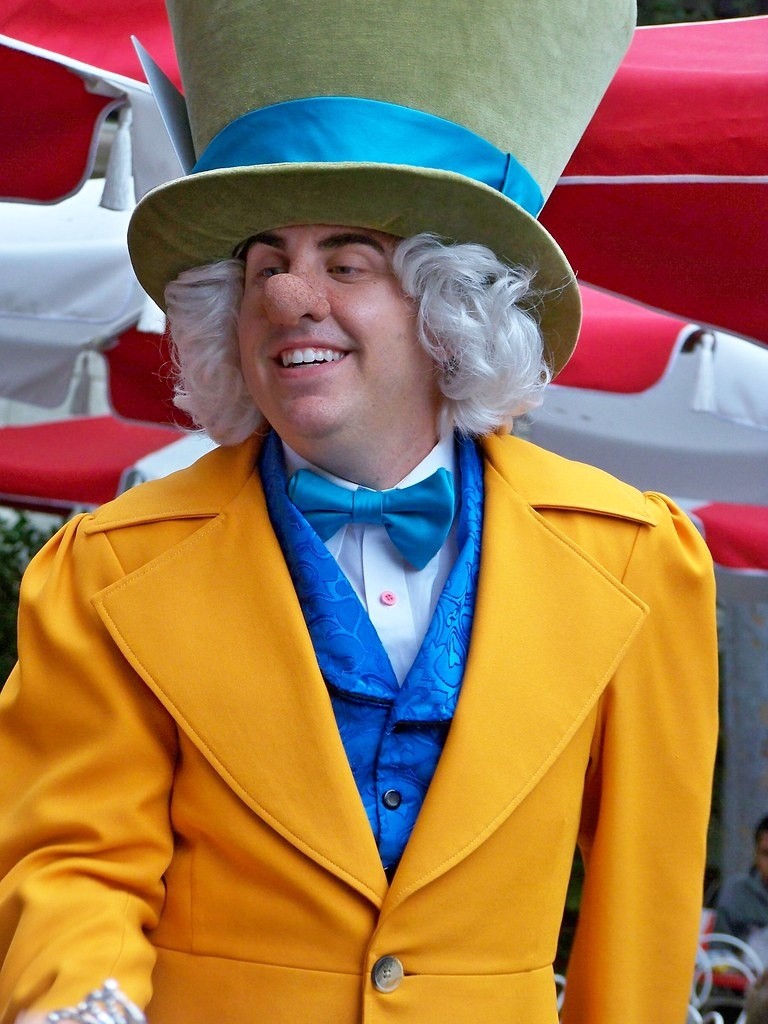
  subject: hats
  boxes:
[124,0,638,382]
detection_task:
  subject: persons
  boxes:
[709,814,768,975]
[0,225,720,1024]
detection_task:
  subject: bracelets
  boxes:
[45,977,147,1024]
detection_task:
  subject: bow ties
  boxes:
[287,466,455,572]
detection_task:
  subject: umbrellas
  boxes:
[0,0,767,575]
